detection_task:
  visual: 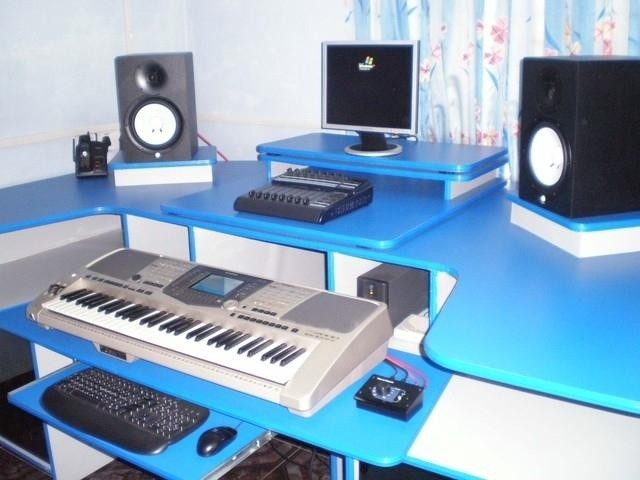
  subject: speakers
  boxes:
[518,54,640,217]
[115,52,198,161]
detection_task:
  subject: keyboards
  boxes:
[41,366,209,454]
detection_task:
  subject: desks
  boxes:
[0,160,640,480]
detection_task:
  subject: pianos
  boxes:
[26,248,395,417]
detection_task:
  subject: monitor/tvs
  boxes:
[321,39,419,157]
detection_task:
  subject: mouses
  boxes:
[197,426,237,455]
